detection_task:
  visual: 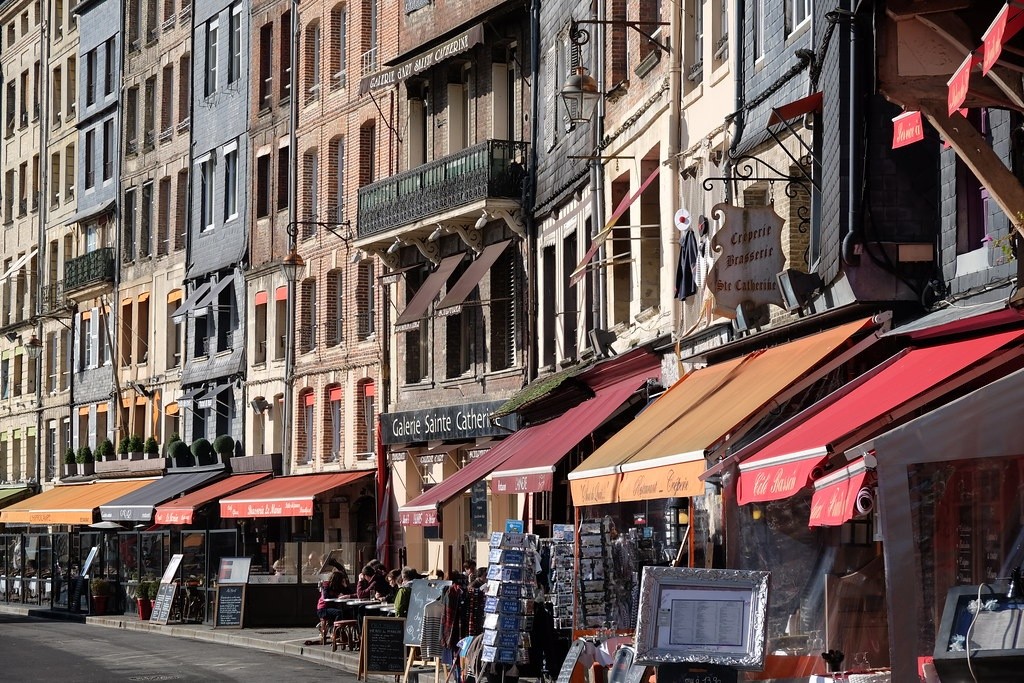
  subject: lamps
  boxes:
[91,579,112,615]
[24,315,71,359]
[735,300,765,332]
[776,269,821,315]
[560,15,671,123]
[250,396,272,415]
[589,329,618,356]
[132,384,149,397]
[4,331,22,342]
[278,220,350,286]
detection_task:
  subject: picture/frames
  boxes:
[161,553,184,584]
[217,557,252,584]
[633,566,772,672]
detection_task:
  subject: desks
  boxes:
[324,599,395,631]
[809,668,891,683]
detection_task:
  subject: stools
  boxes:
[332,620,361,652]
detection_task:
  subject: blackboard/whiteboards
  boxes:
[214,584,246,629]
[556,640,585,683]
[609,648,634,683]
[149,583,177,625]
[623,662,648,683]
[364,616,407,682]
[403,579,452,645]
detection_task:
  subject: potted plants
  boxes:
[148,579,161,607]
[75,448,82,474]
[127,434,144,459]
[80,447,93,474]
[143,434,159,460]
[135,582,150,620]
[100,437,115,461]
[116,436,130,460]
[64,448,76,475]
[168,440,189,468]
[191,438,212,466]
[213,434,234,463]
[93,445,101,473]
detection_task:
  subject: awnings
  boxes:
[569,311,1022,529]
[397,366,652,526]
[98,470,374,525]
[0,487,29,502]
[0,475,162,525]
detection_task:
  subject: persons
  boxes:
[318,559,491,641]
